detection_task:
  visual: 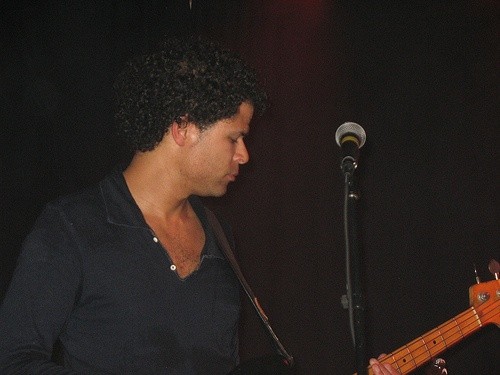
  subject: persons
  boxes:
[0,31,403,375]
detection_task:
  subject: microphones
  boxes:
[335,122,366,182]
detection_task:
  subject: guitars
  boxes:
[353,257,500,375]
